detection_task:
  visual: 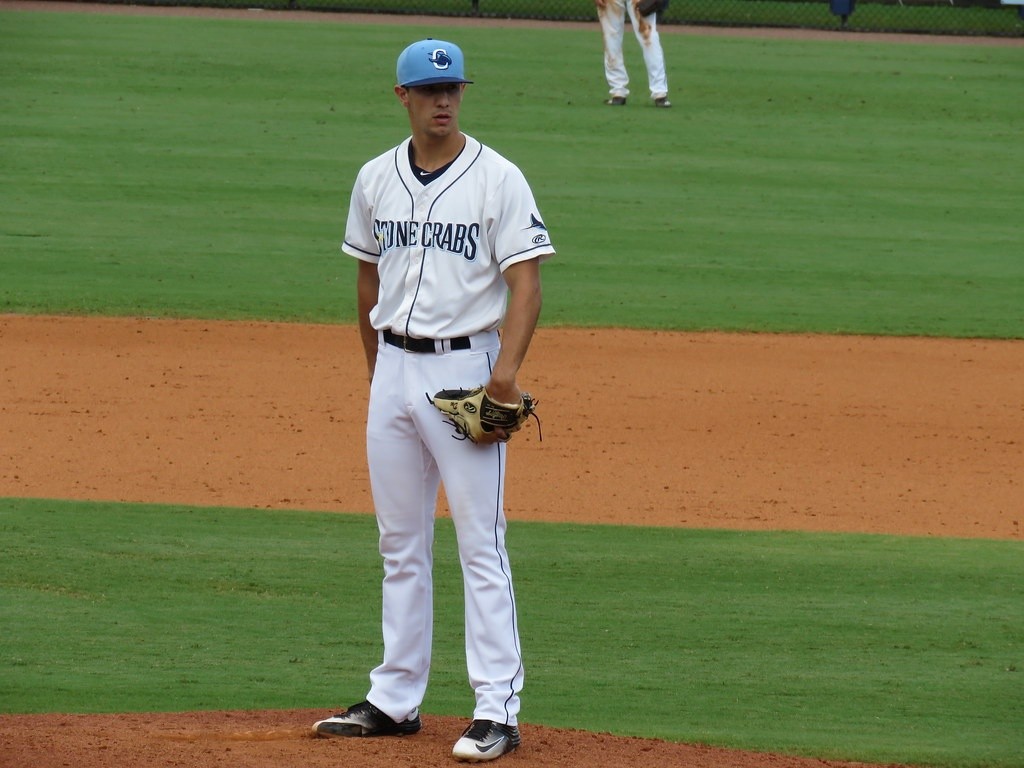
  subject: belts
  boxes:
[383,330,500,354]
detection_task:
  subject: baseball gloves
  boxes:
[433,389,534,446]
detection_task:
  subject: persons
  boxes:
[593,0,672,109]
[310,36,556,760]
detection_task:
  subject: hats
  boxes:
[397,38,474,89]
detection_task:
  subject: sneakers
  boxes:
[451,719,520,763]
[311,700,422,736]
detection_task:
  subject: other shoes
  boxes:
[606,96,627,104]
[656,97,672,107]
